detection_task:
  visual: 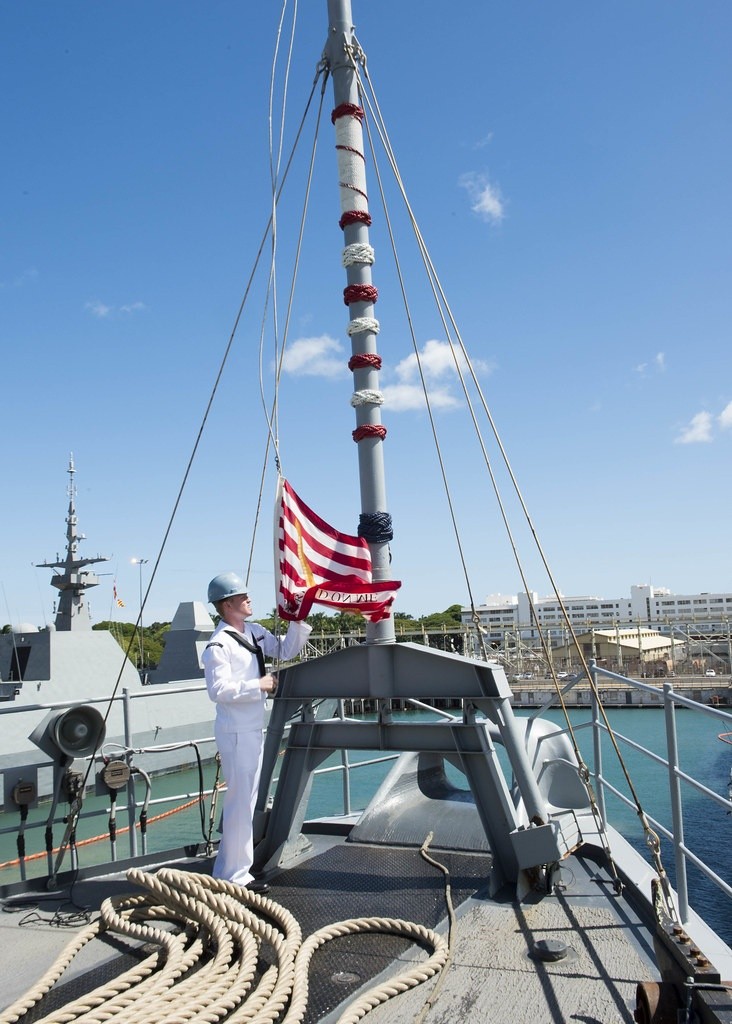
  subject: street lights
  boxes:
[131,558,149,671]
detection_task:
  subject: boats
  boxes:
[1,451,342,817]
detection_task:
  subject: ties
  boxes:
[224,628,266,679]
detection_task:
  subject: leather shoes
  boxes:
[245,882,271,894]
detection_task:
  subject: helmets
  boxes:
[207,572,248,602]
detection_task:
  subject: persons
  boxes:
[201,574,317,894]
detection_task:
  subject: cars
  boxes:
[667,671,676,677]
[705,668,716,677]
[504,670,578,683]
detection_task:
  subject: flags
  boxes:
[272,472,403,621]
[112,579,125,607]
